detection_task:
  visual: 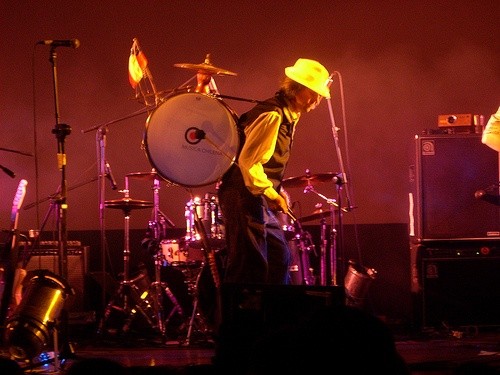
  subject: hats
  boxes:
[284,57,332,99]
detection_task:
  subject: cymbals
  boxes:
[172,52,238,79]
[281,167,339,190]
[102,197,154,209]
[297,202,358,223]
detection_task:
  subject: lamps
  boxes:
[2,269,76,369]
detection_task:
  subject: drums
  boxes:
[143,89,247,191]
[183,192,228,250]
[160,237,217,289]
[17,240,90,318]
[280,222,317,286]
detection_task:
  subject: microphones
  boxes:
[38,39,79,49]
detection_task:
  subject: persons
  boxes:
[217,59,333,286]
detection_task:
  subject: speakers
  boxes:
[409,242,500,344]
[416,133,500,242]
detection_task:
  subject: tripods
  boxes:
[91,174,184,346]
